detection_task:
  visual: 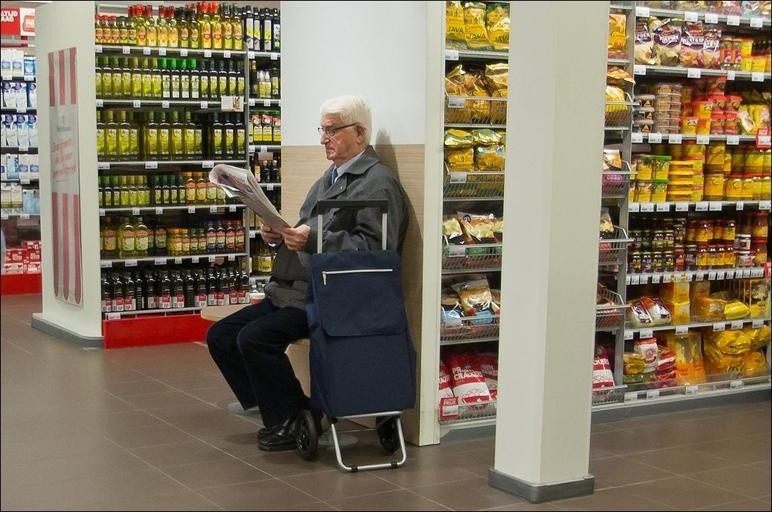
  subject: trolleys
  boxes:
[294,198,417,473]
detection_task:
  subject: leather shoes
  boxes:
[258,410,328,452]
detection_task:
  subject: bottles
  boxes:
[94,0,280,51]
[102,267,249,313]
[95,55,245,101]
[98,166,248,210]
[97,110,246,163]
[250,56,280,293]
[100,217,245,259]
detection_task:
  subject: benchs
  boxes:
[199,301,320,347]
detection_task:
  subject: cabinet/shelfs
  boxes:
[0,40,43,296]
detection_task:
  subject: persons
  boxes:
[207,94,409,451]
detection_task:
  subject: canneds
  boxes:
[626,209,769,274]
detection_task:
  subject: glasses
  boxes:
[317,121,359,136]
